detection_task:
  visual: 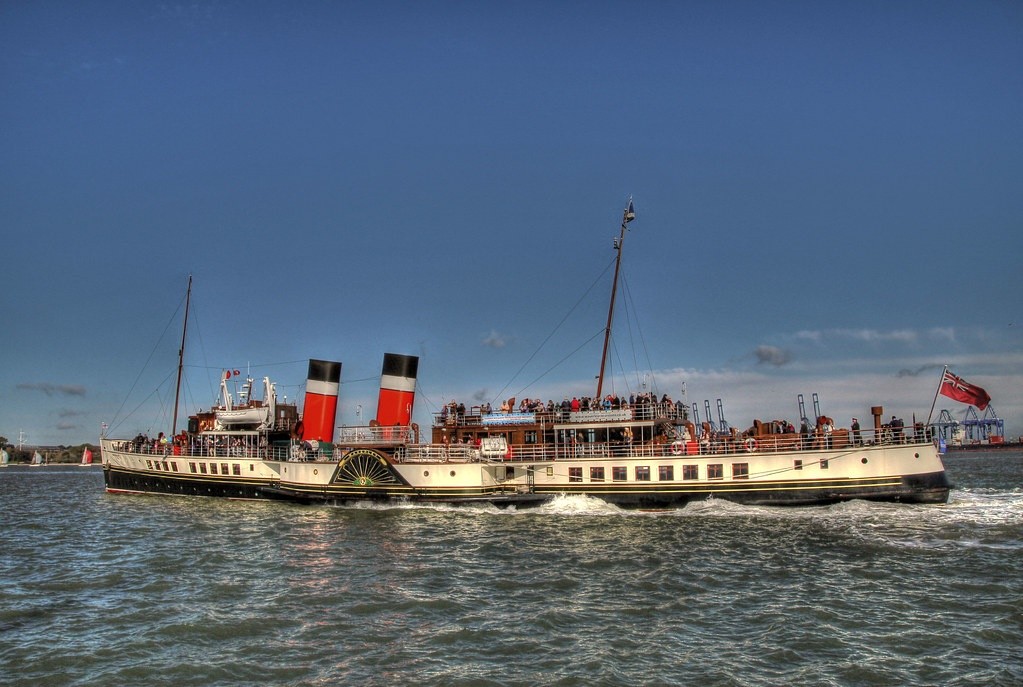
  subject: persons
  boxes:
[691,415,932,455]
[401,393,692,462]
[317,435,323,442]
[298,439,348,465]
[130,429,271,460]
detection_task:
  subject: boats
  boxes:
[98,187,949,505]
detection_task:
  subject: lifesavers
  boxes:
[670,441,686,456]
[393,451,400,461]
[744,438,759,453]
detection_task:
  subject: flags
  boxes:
[939,369,992,411]
[233,369,240,375]
[101,421,109,429]
